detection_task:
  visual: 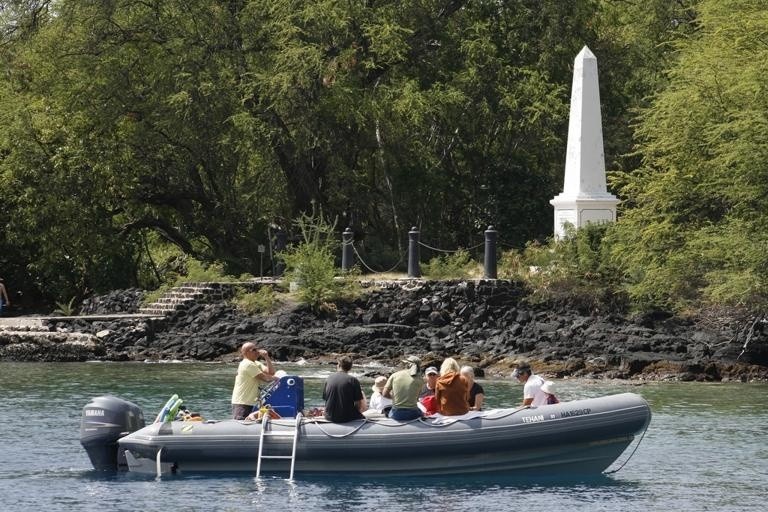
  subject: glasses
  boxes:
[247,347,260,352]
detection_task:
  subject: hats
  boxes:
[425,367,438,374]
[402,356,422,370]
[372,376,387,392]
[337,356,352,371]
[512,367,531,377]
[540,381,556,395]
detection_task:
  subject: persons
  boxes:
[458,365,483,413]
[417,365,439,402]
[369,376,394,413]
[0,278,10,317]
[322,356,368,424]
[433,356,471,416]
[381,355,425,421]
[539,380,559,406]
[230,341,280,421]
[508,360,547,407]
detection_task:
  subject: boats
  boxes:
[75,388,655,480]
